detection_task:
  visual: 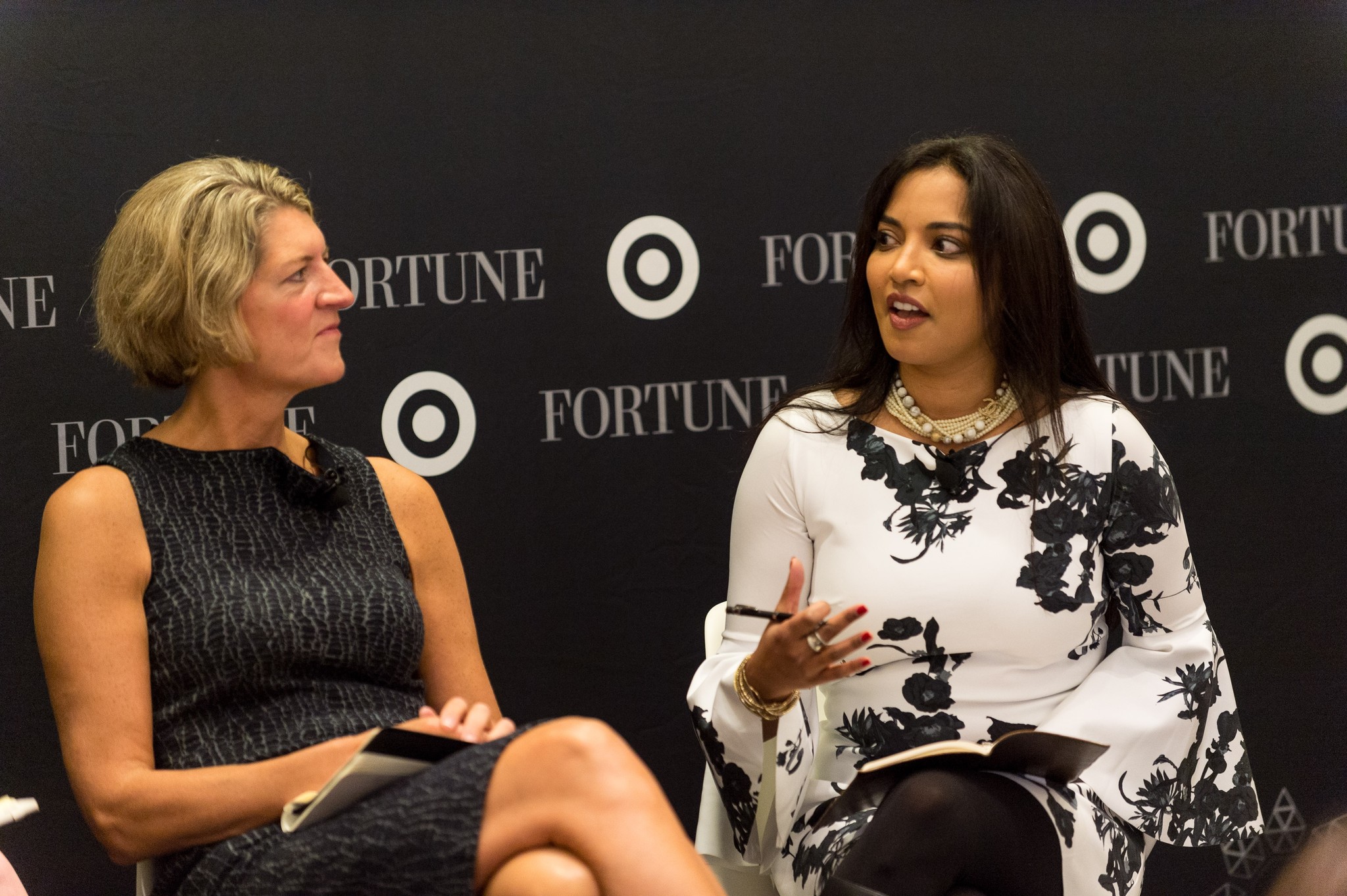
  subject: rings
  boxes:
[806,630,831,653]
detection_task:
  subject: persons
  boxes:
[44,156,733,896]
[684,130,1280,896]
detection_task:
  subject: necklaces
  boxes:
[886,370,1019,445]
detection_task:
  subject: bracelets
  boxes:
[734,656,800,722]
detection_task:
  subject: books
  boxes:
[277,714,483,838]
[811,728,1109,832]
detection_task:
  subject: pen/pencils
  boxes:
[724,604,833,627]
